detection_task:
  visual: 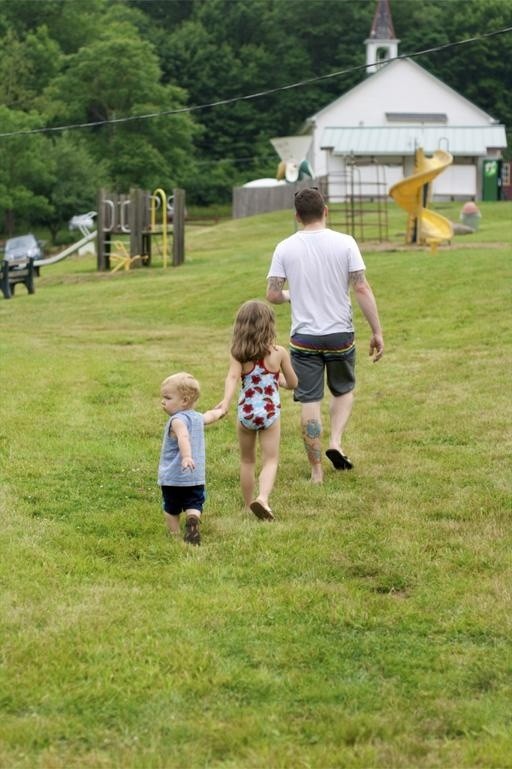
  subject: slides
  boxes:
[389,147,454,246]
[32,229,97,270]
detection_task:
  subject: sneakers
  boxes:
[180,514,203,549]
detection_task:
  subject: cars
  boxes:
[4,233,45,267]
[68,214,95,231]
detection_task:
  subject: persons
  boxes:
[263,186,384,488]
[214,298,299,524]
[157,369,229,548]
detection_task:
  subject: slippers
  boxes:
[250,500,276,523]
[324,448,353,472]
